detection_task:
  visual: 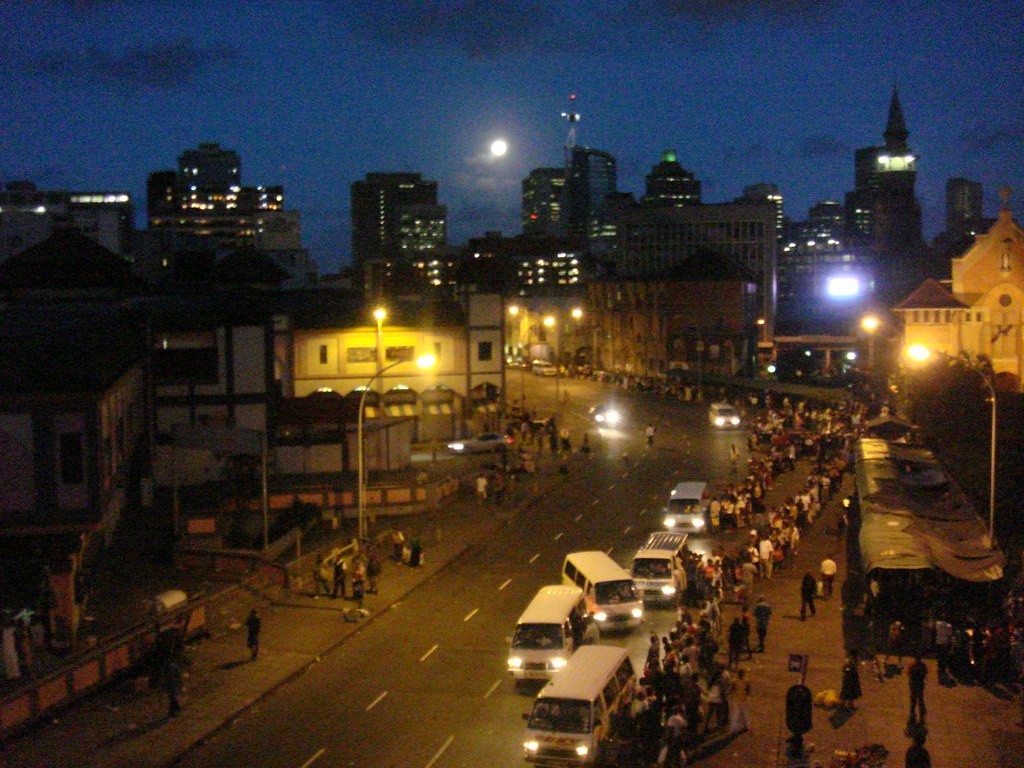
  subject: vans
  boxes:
[504,584,592,687]
[631,531,691,611]
[533,359,557,378]
[661,482,711,537]
[521,644,636,768]
[708,403,741,431]
[561,550,644,637]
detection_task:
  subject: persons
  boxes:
[245,611,262,660]
[617,393,1024,768]
[581,433,592,457]
[391,528,404,563]
[313,560,333,595]
[367,554,383,594]
[645,424,654,446]
[408,531,423,568]
[331,562,346,599]
[352,572,367,609]
[473,405,572,507]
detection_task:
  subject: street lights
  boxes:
[357,349,440,543]
[520,314,557,410]
[903,342,995,541]
